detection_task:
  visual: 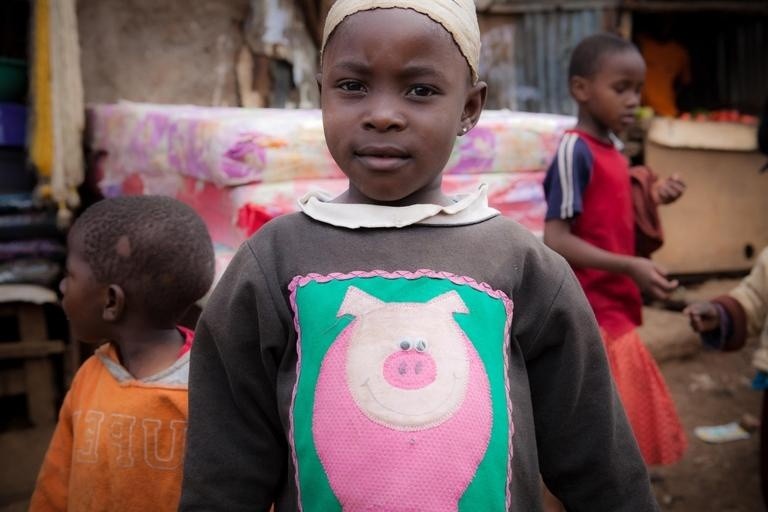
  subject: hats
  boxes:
[320,0,480,86]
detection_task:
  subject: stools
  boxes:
[1,285,65,427]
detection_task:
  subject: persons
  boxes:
[635,14,696,118]
[25,193,218,511]
[536,31,693,510]
[171,2,658,511]
[681,240,766,434]
